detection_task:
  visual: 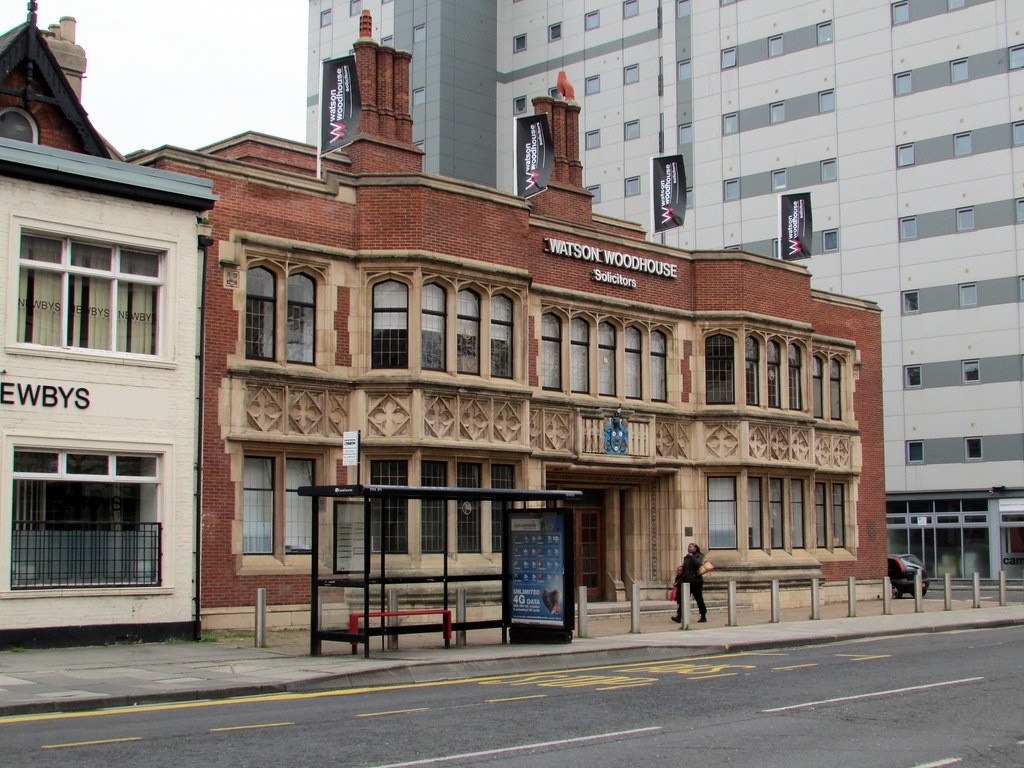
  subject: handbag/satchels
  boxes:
[698,562,714,575]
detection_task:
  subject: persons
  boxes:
[671,543,708,624]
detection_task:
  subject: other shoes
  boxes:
[671,616,681,623]
[697,618,707,622]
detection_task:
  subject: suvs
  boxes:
[887,554,930,599]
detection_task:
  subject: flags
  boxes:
[652,154,687,232]
[516,113,554,201]
[321,54,361,155]
[780,192,813,262]
